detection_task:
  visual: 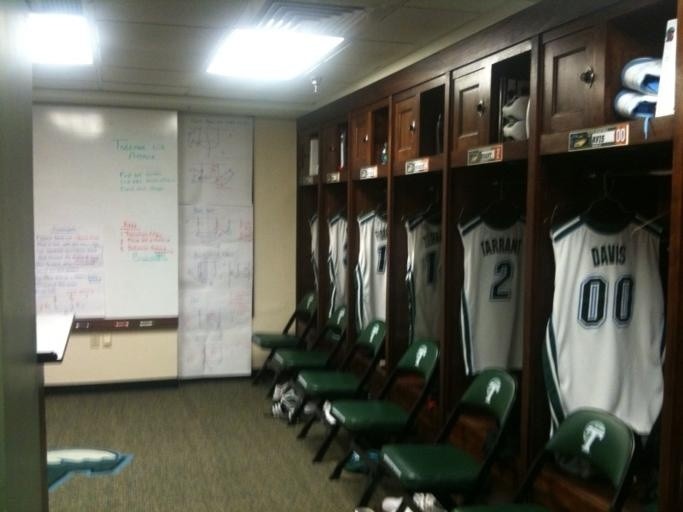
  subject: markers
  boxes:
[136,320,156,328]
[74,322,95,329]
[114,322,133,329]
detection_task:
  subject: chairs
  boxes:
[310,338,443,484]
[251,291,319,398]
[513,406,639,509]
[270,303,349,425]
[352,366,524,509]
[297,317,392,438]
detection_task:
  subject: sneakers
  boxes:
[614,90,658,120]
[621,58,663,95]
[502,121,528,142]
[502,97,527,120]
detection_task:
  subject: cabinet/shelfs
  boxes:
[298,1,681,509]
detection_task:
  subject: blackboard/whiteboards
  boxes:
[32,104,181,320]
